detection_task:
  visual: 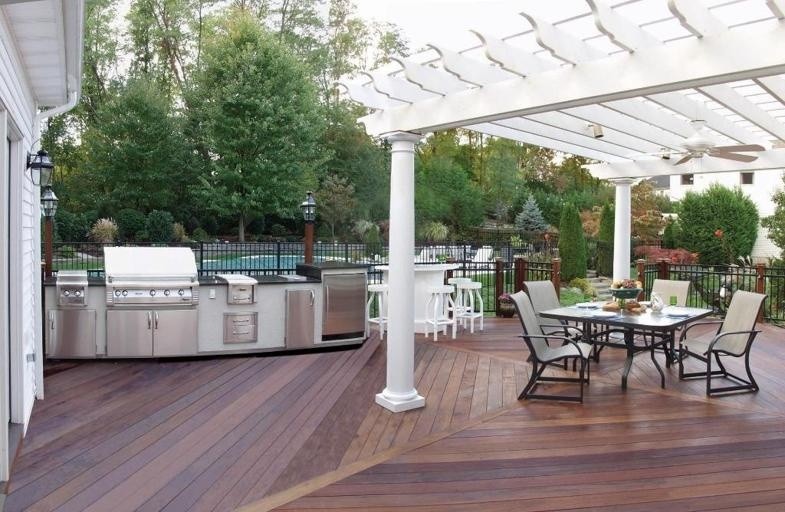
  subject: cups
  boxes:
[670,295,678,307]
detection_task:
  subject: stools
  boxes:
[366,278,484,342]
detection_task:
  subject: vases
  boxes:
[500,302,516,318]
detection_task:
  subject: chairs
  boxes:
[508,290,593,404]
[652,279,691,364]
[679,290,768,398]
[523,280,594,372]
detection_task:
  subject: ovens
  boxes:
[216,273,259,345]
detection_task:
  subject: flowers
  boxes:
[498,292,512,303]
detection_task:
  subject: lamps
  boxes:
[40,188,59,217]
[660,147,671,160]
[300,191,317,221]
[27,137,55,187]
[588,124,604,139]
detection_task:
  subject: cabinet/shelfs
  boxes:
[223,312,257,344]
[105,308,198,358]
[286,288,315,349]
[47,308,97,359]
[323,273,366,341]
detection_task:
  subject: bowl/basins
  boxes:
[607,288,644,300]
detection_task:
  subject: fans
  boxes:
[631,120,766,166]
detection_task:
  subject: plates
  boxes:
[576,301,690,321]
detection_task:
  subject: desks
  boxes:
[375,263,459,334]
[539,301,713,395]
